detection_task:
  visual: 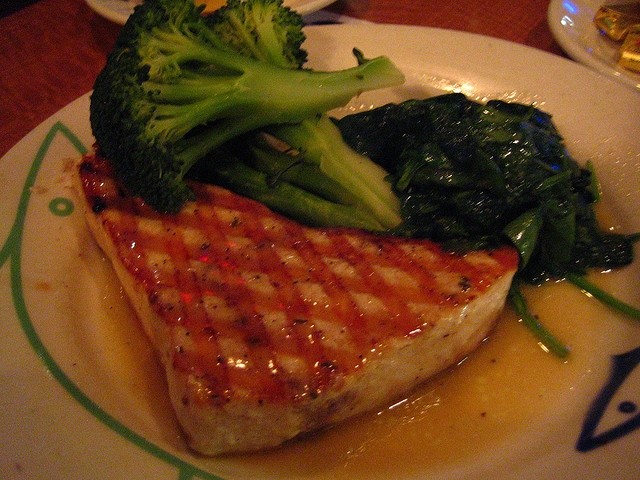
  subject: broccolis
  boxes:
[87,1,405,218]
[185,0,407,234]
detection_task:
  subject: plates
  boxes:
[547,0,640,92]
[85,0,335,24]
[1,23,640,480]
